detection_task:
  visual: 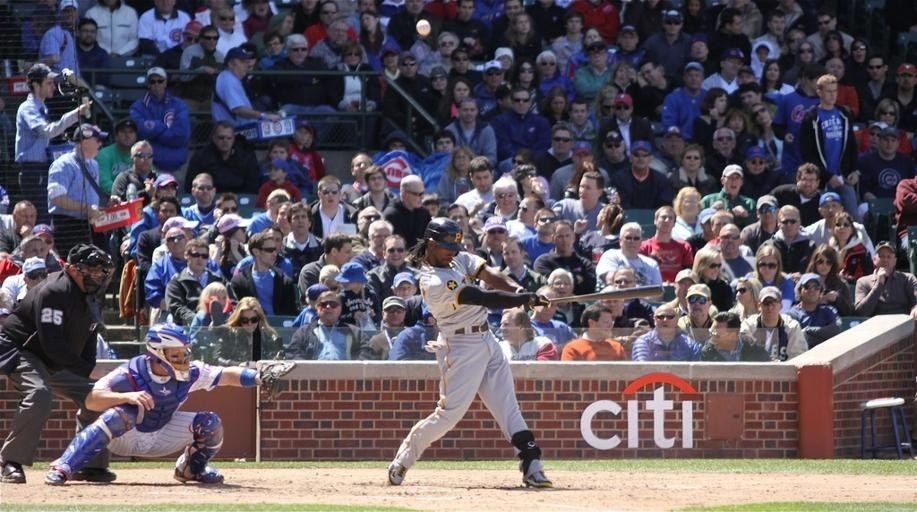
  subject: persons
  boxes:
[386,216,556,489]
[42,322,295,485]
[1,241,116,485]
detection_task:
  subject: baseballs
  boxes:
[417,20,430,34]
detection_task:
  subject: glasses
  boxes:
[26,272,46,279]
[44,239,53,245]
[707,328,733,339]
[133,0,897,326]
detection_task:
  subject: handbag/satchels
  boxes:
[0,325,22,375]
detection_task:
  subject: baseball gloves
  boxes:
[258,360,298,404]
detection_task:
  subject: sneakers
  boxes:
[0,461,26,483]
[67,466,116,483]
[172,464,224,484]
[387,443,408,486]
[521,459,552,487]
[44,466,65,486]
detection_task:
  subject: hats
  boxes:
[58,0,78,14]
[22,257,46,275]
[896,63,916,78]
[27,63,60,80]
[71,123,110,143]
[31,224,53,238]
[114,118,137,137]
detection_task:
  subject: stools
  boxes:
[857,392,917,466]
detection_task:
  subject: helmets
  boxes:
[67,244,115,270]
[143,321,193,382]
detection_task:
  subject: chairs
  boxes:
[175,190,309,363]
[104,50,156,110]
[619,195,916,346]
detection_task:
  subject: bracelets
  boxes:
[240,367,255,387]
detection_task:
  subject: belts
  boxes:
[455,321,487,334]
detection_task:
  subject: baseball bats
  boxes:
[528,285,664,310]
